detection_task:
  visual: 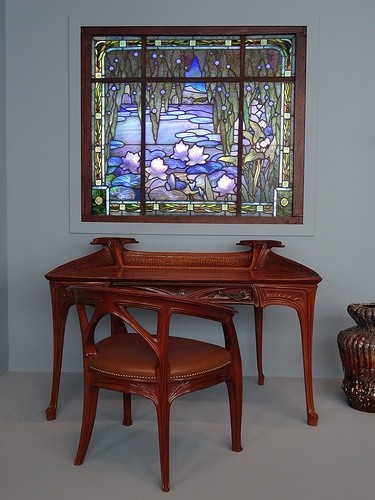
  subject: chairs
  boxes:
[63,286,244,493]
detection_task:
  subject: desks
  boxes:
[43,237,322,427]
